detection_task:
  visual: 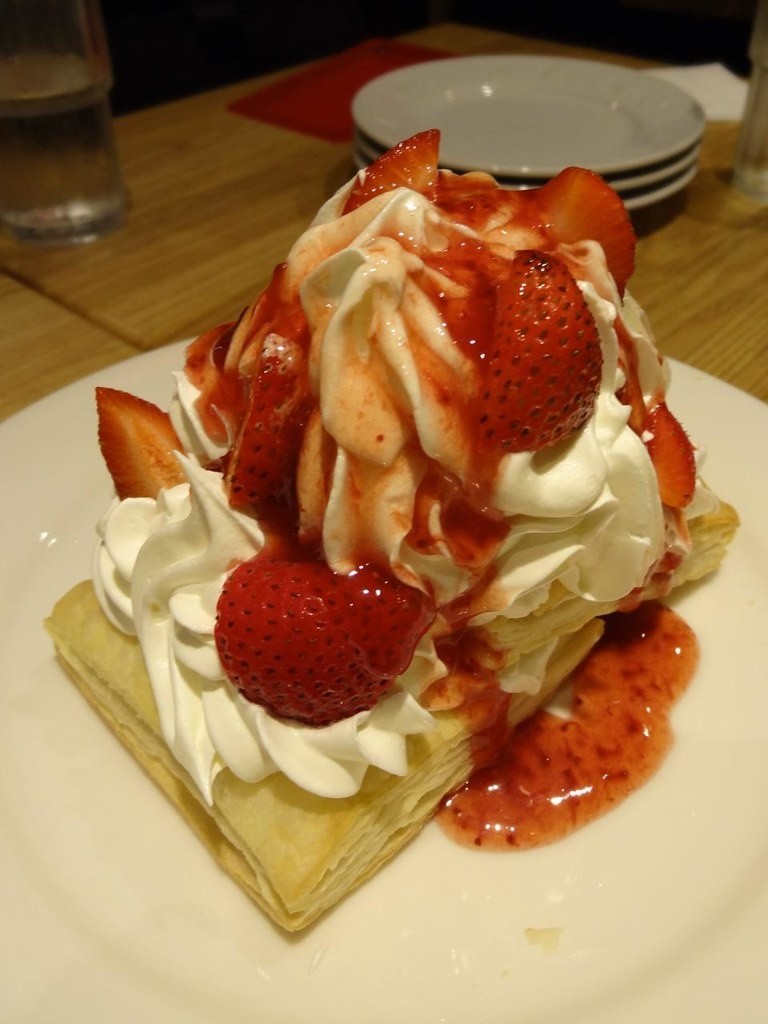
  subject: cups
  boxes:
[727,1,768,201]
[0,0,129,246]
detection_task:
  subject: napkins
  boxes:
[644,62,749,121]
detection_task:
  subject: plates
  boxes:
[351,53,705,211]
[1,340,768,1022]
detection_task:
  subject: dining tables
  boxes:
[0,19,768,421]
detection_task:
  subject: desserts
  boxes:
[41,127,744,936]
